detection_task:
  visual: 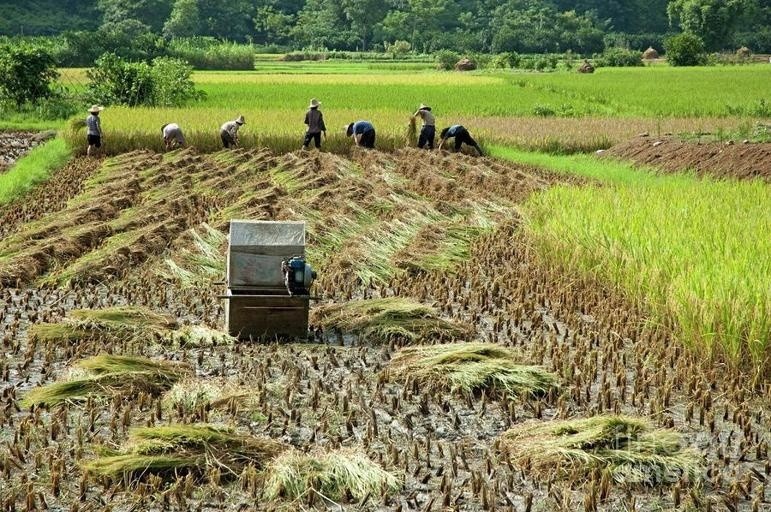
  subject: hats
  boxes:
[88,105,104,113]
[441,128,453,138]
[308,98,320,108]
[418,103,431,111]
[344,122,354,137]
[161,123,168,132]
[236,115,245,124]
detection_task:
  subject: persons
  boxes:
[220,115,248,149]
[409,105,436,150]
[344,120,376,148]
[439,125,484,157]
[302,98,328,151]
[162,123,188,151]
[86,105,104,159]
